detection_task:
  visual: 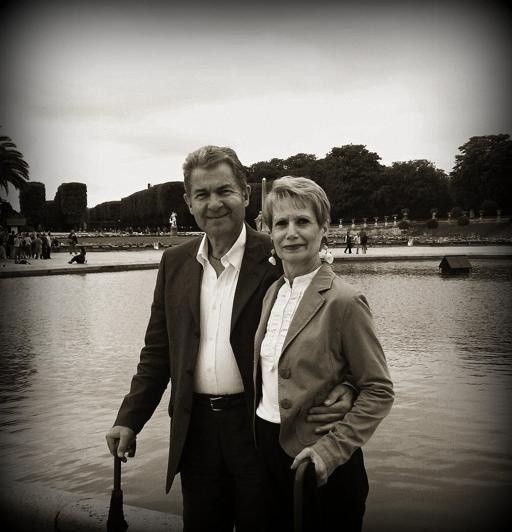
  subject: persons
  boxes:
[105,146,360,532]
[344,229,368,254]
[252,176,395,532]
[0,229,86,264]
[169,212,177,225]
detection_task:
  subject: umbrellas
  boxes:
[293,458,313,532]
[106,438,136,532]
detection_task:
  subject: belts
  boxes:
[192,394,247,412]
